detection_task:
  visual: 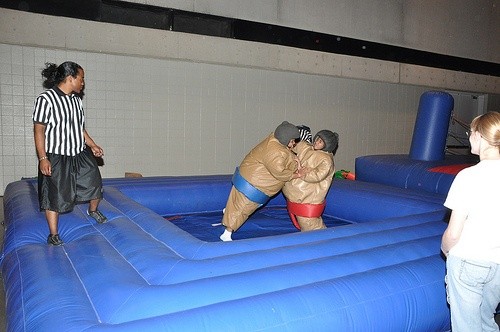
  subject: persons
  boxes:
[281,130,338,233]
[33,61,108,246]
[440,111,500,332]
[219,123,308,242]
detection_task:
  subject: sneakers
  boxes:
[88,208,108,224]
[47,234,63,245]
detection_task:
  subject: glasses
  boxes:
[466,132,477,136]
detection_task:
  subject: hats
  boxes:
[313,130,339,153]
[275,121,299,146]
[294,125,312,143]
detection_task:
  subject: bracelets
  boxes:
[37,155,49,162]
